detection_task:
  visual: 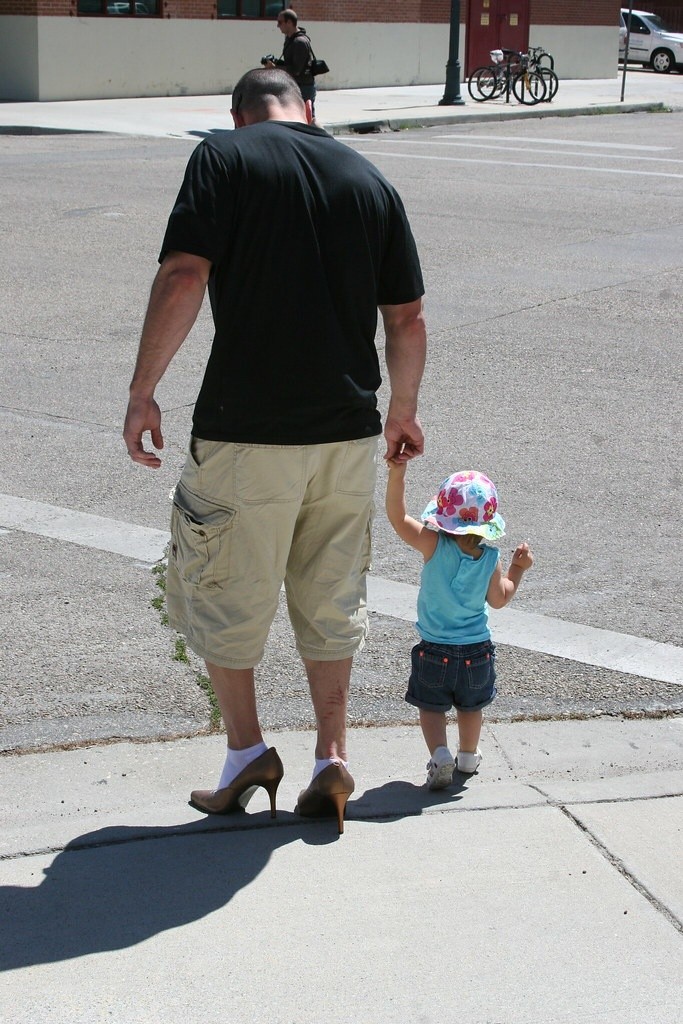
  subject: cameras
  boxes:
[261,55,274,66]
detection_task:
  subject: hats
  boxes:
[420,470,507,542]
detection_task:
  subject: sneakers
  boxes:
[424,746,455,790]
[456,745,483,774]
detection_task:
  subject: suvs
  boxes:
[620,7,683,75]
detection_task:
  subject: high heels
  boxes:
[190,746,284,818]
[298,760,355,834]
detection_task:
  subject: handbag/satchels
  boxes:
[274,58,329,77]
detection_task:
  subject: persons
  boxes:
[260,9,318,110]
[122,68,425,832]
[384,451,534,791]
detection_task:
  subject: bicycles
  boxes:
[467,51,547,106]
[476,46,559,102]
